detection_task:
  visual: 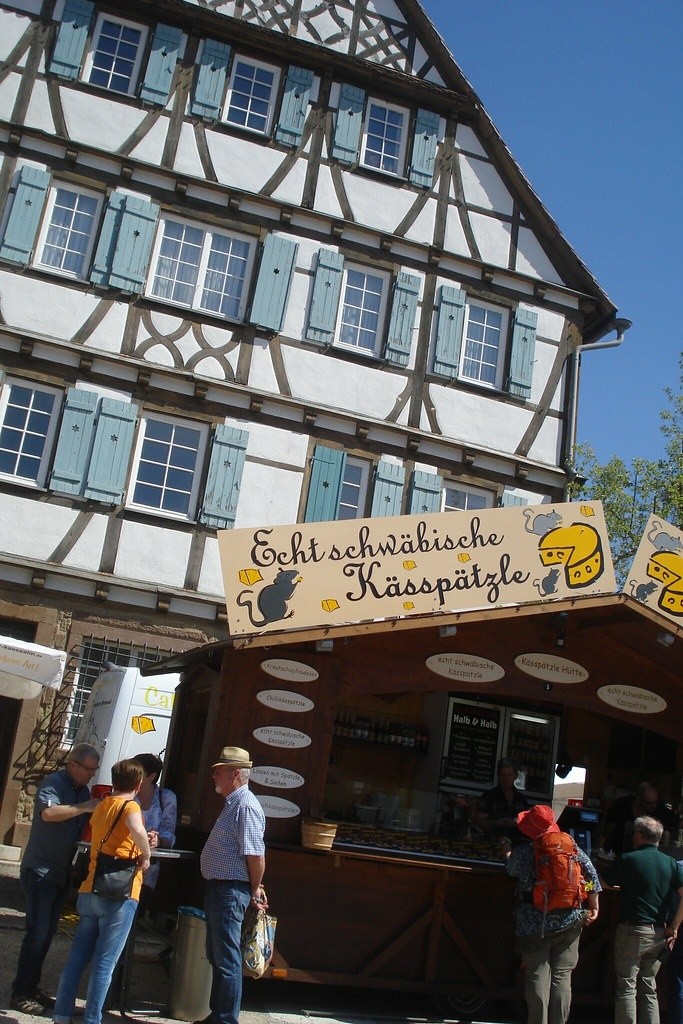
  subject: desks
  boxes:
[66,842,180,1024]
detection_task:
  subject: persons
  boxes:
[51,758,152,1024]
[605,782,683,855]
[132,753,178,920]
[9,743,102,1014]
[600,815,683,1024]
[473,757,530,854]
[503,804,604,1024]
[192,746,269,1024]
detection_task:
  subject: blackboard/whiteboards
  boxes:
[441,699,558,804]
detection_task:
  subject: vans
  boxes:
[67,660,182,860]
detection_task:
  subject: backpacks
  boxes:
[530,832,588,913]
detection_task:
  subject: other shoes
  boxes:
[9,993,45,1015]
[193,1013,221,1024]
[33,990,57,1007]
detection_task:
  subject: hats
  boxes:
[516,804,561,840]
[211,746,253,770]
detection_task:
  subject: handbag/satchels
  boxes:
[90,851,136,900]
[240,889,278,978]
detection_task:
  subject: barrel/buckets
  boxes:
[300,819,337,850]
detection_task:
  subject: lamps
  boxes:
[439,625,458,637]
[656,632,674,647]
[316,641,334,652]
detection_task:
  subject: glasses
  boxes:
[71,759,99,772]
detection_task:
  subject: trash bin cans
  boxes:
[164,903,214,1021]
